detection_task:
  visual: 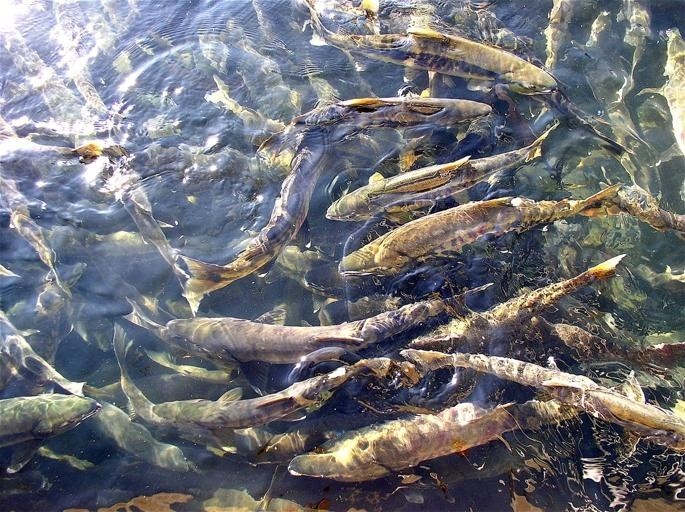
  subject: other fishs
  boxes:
[0,0,685,512]
